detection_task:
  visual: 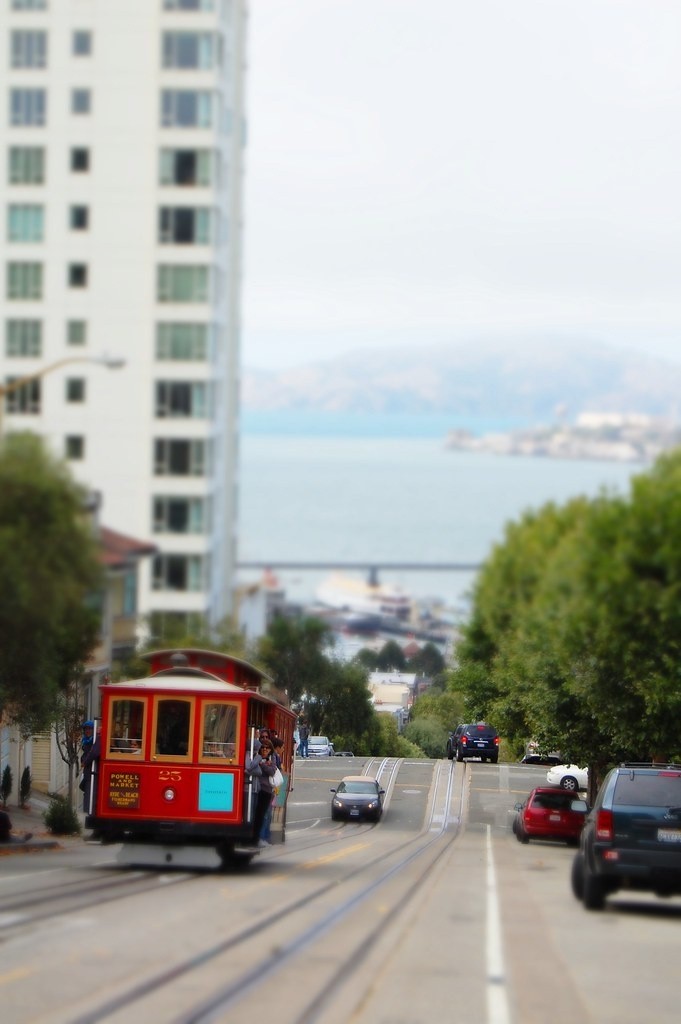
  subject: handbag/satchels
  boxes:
[269,765,283,787]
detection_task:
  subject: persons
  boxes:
[131,737,142,755]
[79,720,95,812]
[298,719,311,760]
[81,726,102,817]
[82,827,102,842]
[247,726,285,851]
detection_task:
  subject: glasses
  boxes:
[261,735,268,738]
[262,745,270,749]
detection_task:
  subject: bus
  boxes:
[87,644,298,866]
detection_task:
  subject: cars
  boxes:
[329,775,386,820]
[336,752,355,757]
[304,736,335,757]
[512,786,584,845]
[520,754,561,765]
[546,761,590,791]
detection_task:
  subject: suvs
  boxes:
[445,723,499,762]
[568,762,681,911]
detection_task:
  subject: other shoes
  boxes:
[258,839,271,847]
